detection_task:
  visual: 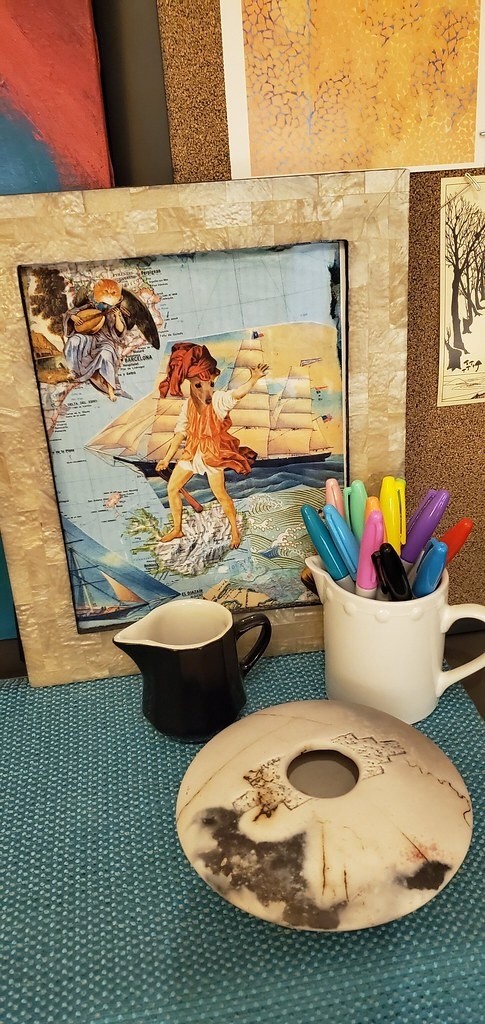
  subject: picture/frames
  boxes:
[0,166,410,687]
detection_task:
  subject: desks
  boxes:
[0,631,485,1024]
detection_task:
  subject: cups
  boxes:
[112,598,271,746]
[305,551,485,728]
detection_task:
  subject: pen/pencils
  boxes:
[411,537,448,600]
[356,511,384,600]
[321,505,361,582]
[372,543,412,605]
[378,476,408,560]
[325,478,346,518]
[300,505,354,592]
[398,490,450,574]
[438,517,473,566]
[364,496,379,520]
[343,480,368,545]
